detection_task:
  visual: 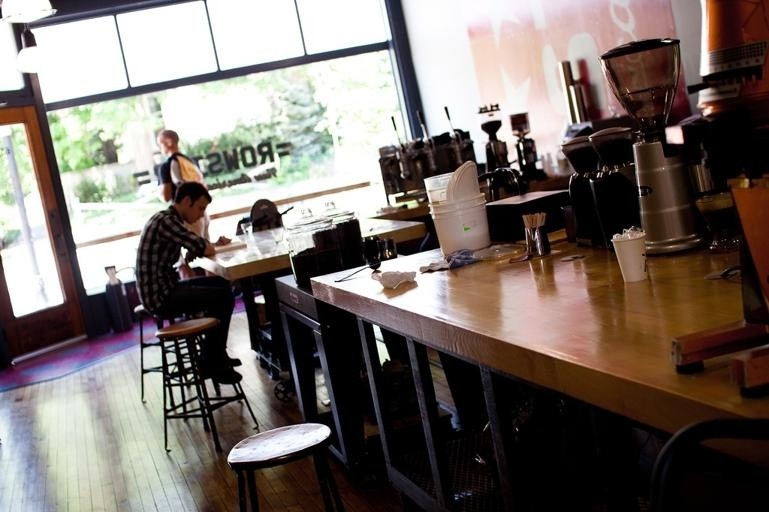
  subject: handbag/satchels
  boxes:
[170,155,204,188]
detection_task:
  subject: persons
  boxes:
[134,181,243,384]
[157,129,211,320]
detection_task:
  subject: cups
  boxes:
[270,227,283,243]
[241,222,253,236]
[611,232,648,283]
[525,226,551,258]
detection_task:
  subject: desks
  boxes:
[186,212,427,401]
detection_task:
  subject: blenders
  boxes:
[598,36,707,257]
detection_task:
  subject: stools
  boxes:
[133,304,257,456]
[225,422,346,512]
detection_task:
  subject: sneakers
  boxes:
[200,348,242,385]
